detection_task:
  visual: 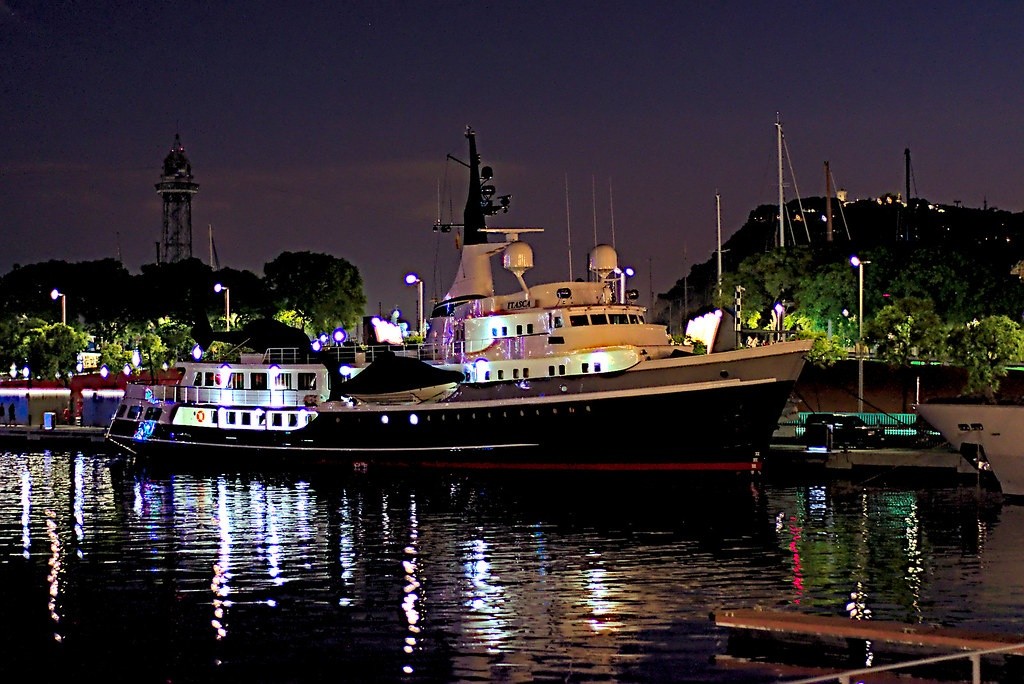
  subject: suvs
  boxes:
[807,412,888,449]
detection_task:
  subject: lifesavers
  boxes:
[197,411,204,422]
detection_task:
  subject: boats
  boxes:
[911,400,1024,496]
[106,348,330,455]
[312,122,817,474]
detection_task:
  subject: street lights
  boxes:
[50,288,68,325]
[614,268,625,304]
[211,282,231,331]
[852,255,864,340]
[404,275,426,340]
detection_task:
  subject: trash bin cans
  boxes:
[44,412,56,430]
